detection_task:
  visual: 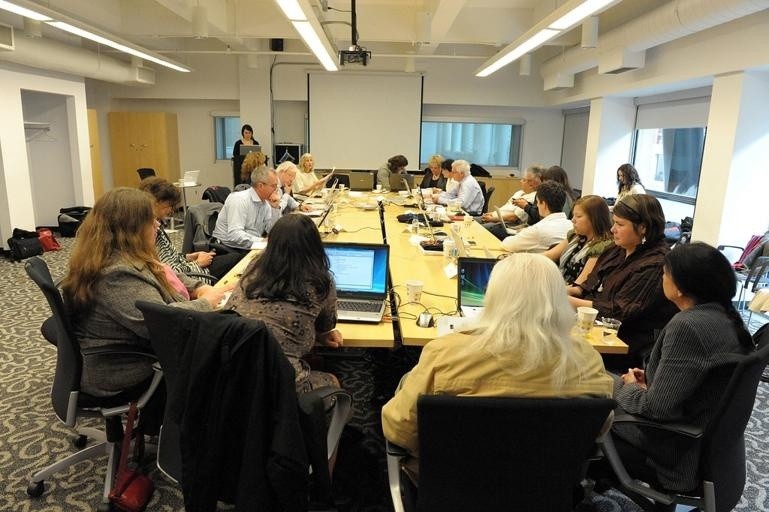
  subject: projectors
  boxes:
[339,49,372,67]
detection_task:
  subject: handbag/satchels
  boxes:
[58,206,92,237]
[108,467,154,511]
[7,228,61,261]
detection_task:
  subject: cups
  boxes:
[178,178,184,187]
[339,184,345,193]
[442,240,457,264]
[406,280,423,305]
[577,306,598,338]
[398,183,474,256]
[323,219,334,233]
[376,185,382,191]
[600,317,622,343]
[329,204,339,217]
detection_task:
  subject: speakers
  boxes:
[272,39,284,52]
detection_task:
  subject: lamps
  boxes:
[473,0,622,78]
[0,0,194,74]
[275,0,340,72]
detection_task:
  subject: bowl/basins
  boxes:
[172,183,180,186]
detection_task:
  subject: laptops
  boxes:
[458,255,498,317]
[182,169,201,186]
[240,145,261,155]
[302,172,515,256]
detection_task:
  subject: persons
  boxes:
[224,213,344,416]
[605,242,754,462]
[211,124,334,253]
[140,177,245,286]
[380,252,614,456]
[377,155,677,370]
[62,186,233,439]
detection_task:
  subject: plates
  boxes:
[372,189,386,194]
[360,203,378,210]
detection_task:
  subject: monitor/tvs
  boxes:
[320,242,391,323]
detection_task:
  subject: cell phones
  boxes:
[209,248,217,254]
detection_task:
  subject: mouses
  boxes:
[417,309,431,328]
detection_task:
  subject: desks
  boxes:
[381,190,630,394]
[171,181,199,227]
[207,186,392,480]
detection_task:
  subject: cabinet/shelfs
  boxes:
[87,109,105,203]
[108,111,181,209]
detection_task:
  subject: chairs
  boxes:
[323,169,378,191]
[382,390,617,481]
[27,257,179,509]
[711,234,768,316]
[600,323,769,509]
[132,301,350,511]
[443,177,493,215]
[185,181,250,290]
